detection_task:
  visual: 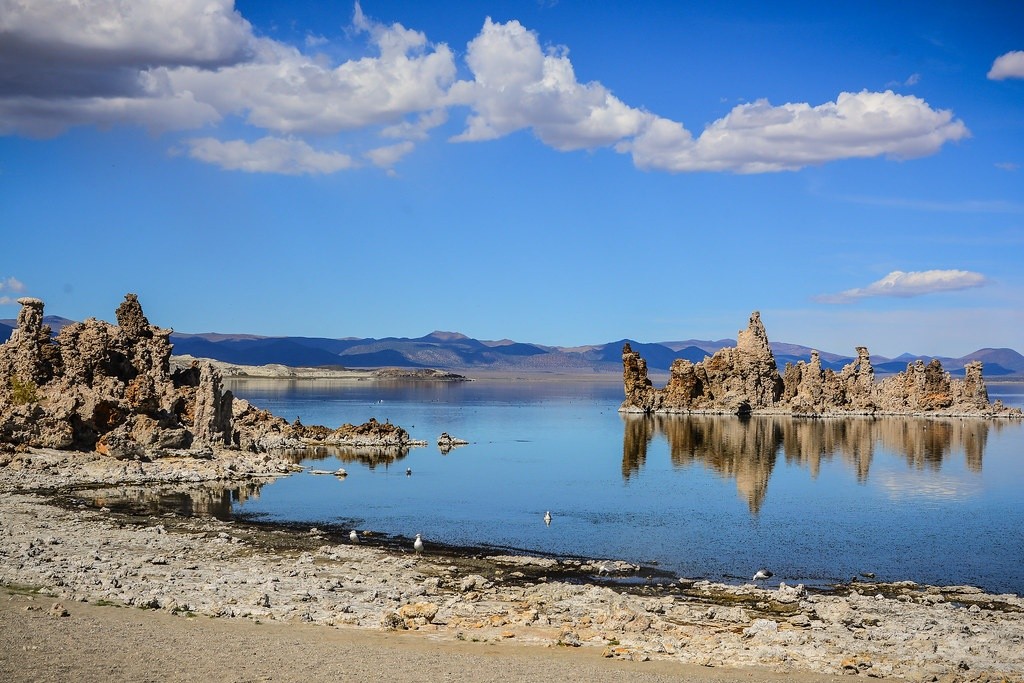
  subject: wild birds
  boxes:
[544,511,552,520]
[405,467,411,476]
[752,569,776,586]
[413,534,424,556]
[923,425,926,430]
[348,530,367,546]
